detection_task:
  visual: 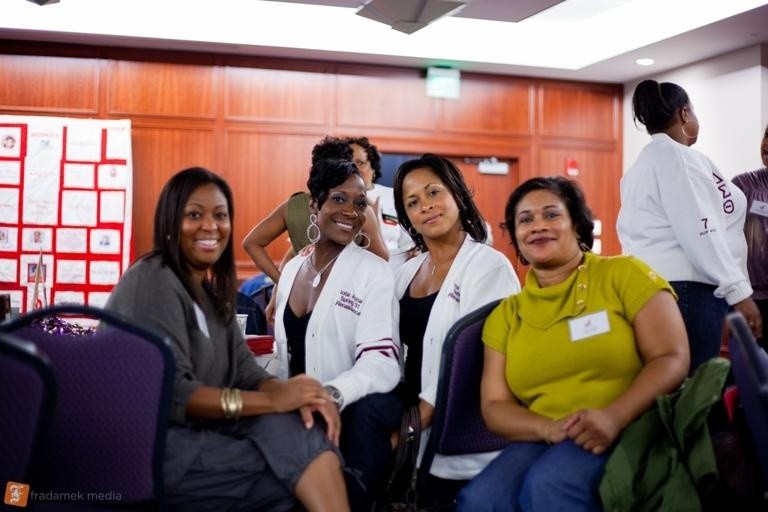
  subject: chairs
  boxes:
[0,299,504,512]
[728,312,768,444]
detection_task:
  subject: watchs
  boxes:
[322,383,347,409]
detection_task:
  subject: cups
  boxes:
[235,313,248,338]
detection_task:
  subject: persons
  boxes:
[100,166,353,512]
[240,135,391,325]
[263,159,406,480]
[340,152,526,512]
[731,122,768,305]
[343,136,414,249]
[447,175,698,512]
[615,78,763,361]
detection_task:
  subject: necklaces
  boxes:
[307,253,338,288]
[427,232,466,276]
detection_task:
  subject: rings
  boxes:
[749,322,753,326]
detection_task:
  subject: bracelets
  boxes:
[542,417,560,444]
[219,387,243,420]
